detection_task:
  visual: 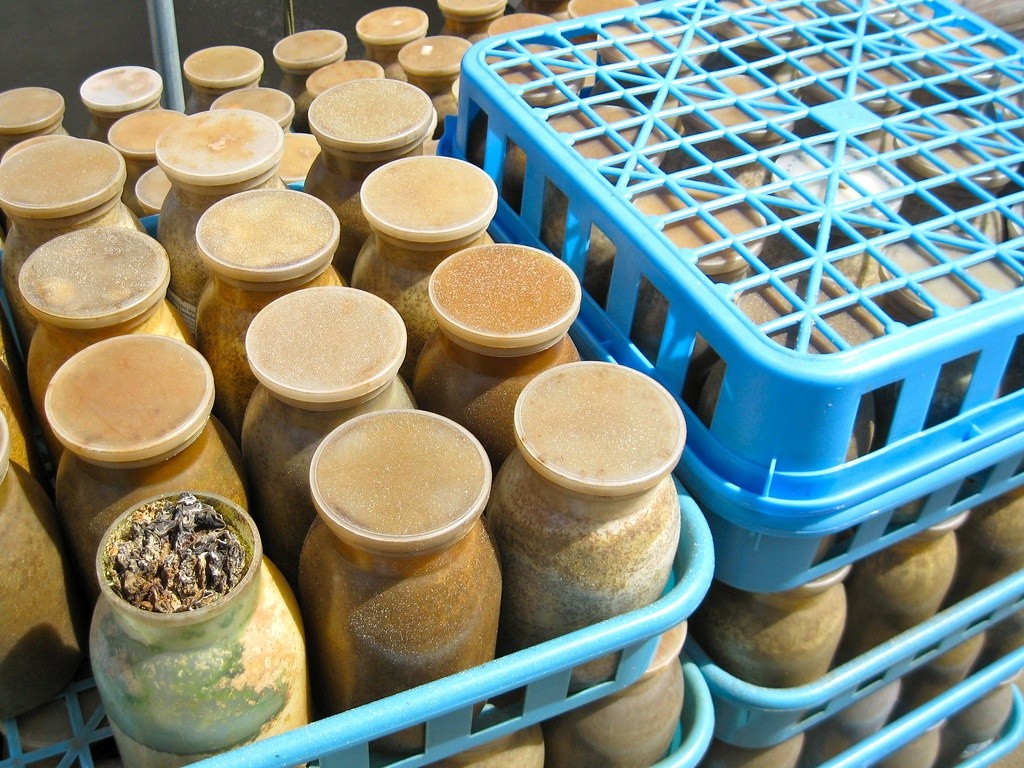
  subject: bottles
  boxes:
[0,0,1024,768]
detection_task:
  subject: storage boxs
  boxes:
[0,0,1024,768]
